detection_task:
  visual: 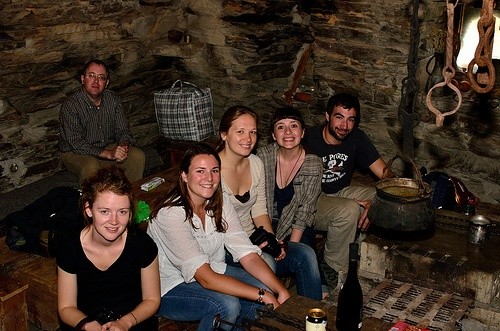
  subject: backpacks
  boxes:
[5,186,87,258]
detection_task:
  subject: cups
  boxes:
[466,214,492,245]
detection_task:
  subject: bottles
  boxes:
[428,51,447,98]
[336,242,363,331]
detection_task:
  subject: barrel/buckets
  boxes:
[375,154,435,243]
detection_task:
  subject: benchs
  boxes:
[0,145,165,234]
[434,202,500,237]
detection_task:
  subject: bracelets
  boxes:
[129,312,138,324]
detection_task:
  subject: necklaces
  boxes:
[278,149,302,188]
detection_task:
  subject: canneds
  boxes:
[306,308,327,323]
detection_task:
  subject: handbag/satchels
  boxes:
[153,79,216,143]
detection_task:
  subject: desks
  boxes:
[158,133,224,166]
[247,295,392,331]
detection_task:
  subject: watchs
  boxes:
[256,288,266,303]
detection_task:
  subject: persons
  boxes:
[58,59,147,188]
[213,105,324,302]
[256,106,332,299]
[146,146,291,331]
[54,166,161,331]
[300,92,397,273]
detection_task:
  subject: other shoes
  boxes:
[318,251,338,293]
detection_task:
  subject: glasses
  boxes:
[85,73,107,81]
